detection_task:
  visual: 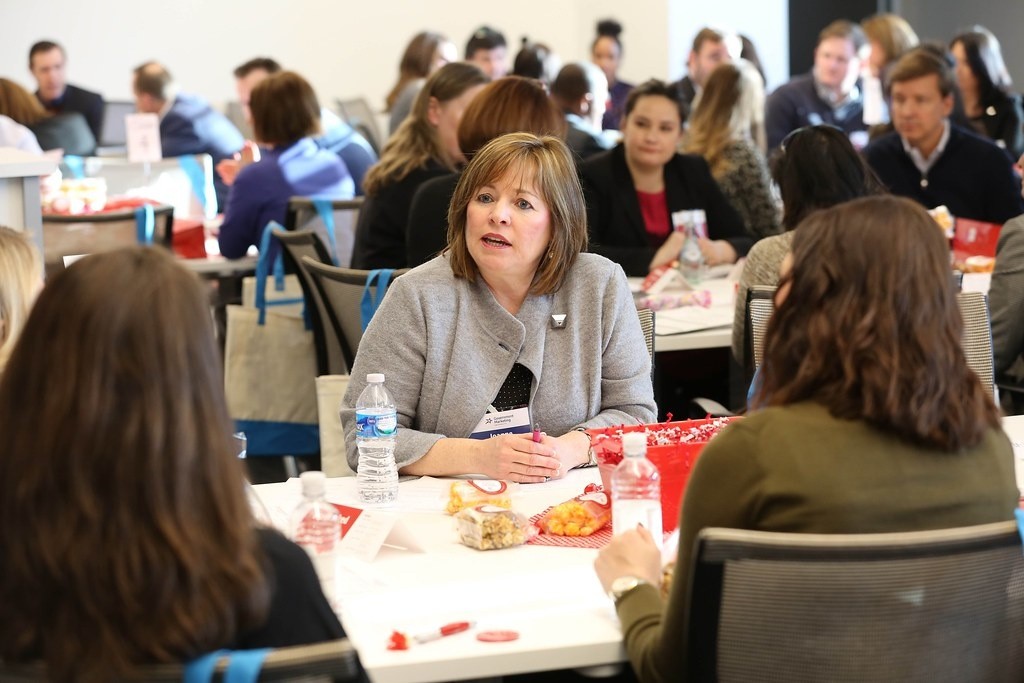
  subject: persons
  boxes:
[0,244,375,683]
[339,133,658,483]
[0,14,1024,413]
[593,197,1018,683]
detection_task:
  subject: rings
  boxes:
[545,476,551,482]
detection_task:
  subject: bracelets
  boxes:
[573,428,598,467]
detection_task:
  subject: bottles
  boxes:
[287,472,340,586]
[612,432,662,545]
[356,374,398,503]
[680,222,701,286]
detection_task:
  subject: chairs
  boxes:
[38,96,1023,683]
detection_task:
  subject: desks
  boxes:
[628,268,992,357]
[186,248,267,337]
[247,412,1024,683]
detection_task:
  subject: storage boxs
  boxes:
[586,415,744,534]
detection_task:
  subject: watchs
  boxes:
[609,575,647,602]
